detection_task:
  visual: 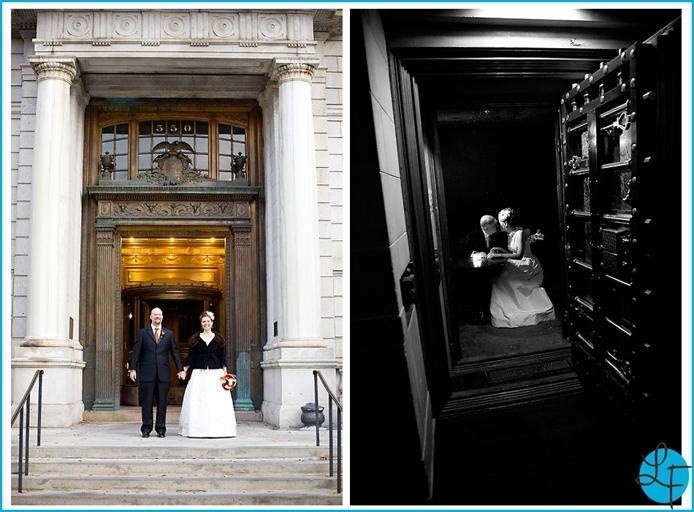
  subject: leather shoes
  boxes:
[142,432,149,437]
[158,432,165,437]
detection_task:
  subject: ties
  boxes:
[154,328,159,342]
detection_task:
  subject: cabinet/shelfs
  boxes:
[558,39,670,446]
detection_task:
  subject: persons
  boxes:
[177,311,237,437]
[453,209,556,328]
[129,308,185,438]
